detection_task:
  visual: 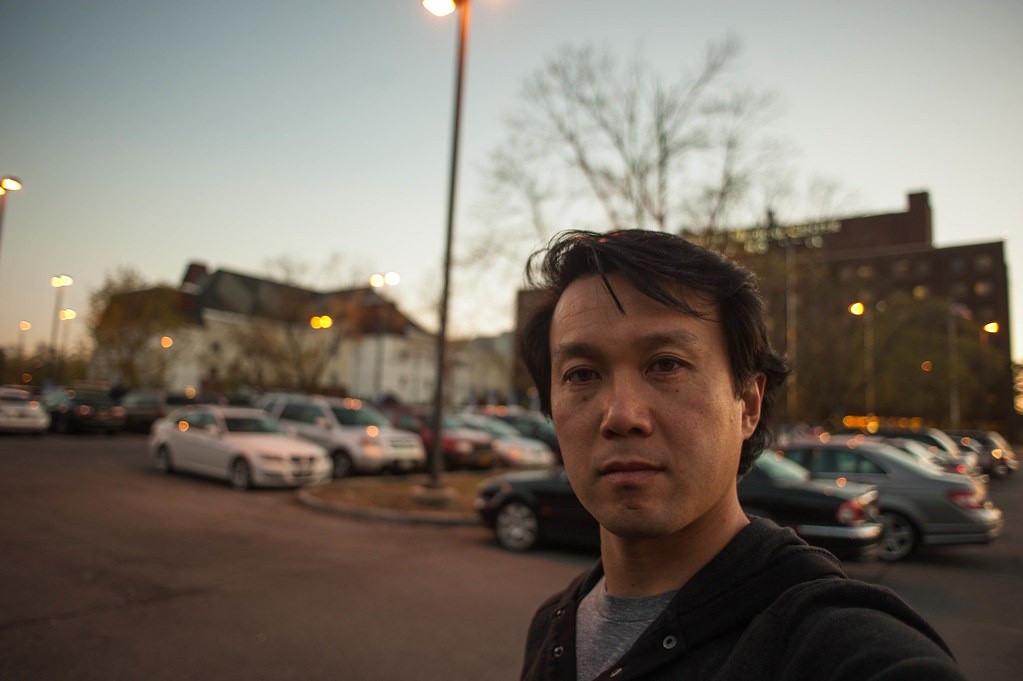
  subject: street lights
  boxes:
[0,175,23,250]
[50,273,73,355]
[415,0,480,505]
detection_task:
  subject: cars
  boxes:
[452,413,558,474]
[0,387,51,434]
[149,404,334,493]
[472,448,888,565]
[36,384,129,434]
[767,434,1007,563]
[853,430,1017,486]
[380,401,496,469]
[502,414,561,462]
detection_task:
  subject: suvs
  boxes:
[109,382,170,434]
[255,392,428,480]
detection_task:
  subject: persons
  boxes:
[519,228,964,681]
